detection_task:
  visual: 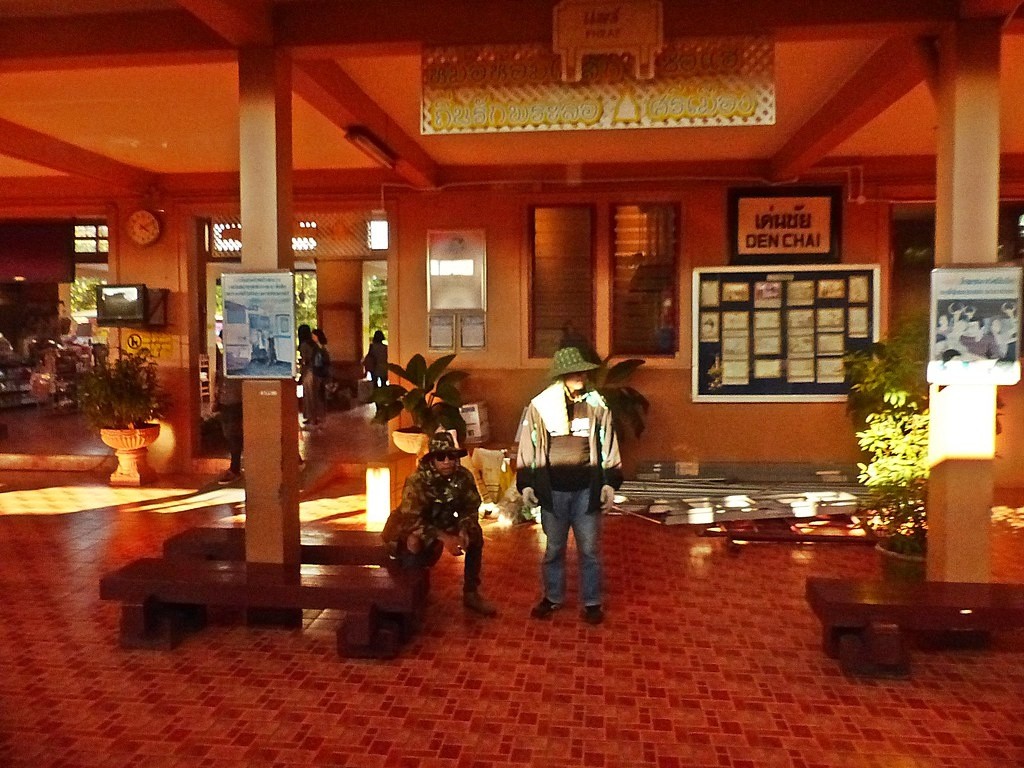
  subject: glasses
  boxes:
[435,454,455,462]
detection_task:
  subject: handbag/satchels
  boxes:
[363,356,376,371]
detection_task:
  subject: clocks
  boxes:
[125,209,163,249]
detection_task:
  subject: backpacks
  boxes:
[308,342,330,377]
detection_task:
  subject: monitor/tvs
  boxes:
[96,284,148,323]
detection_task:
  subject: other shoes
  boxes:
[462,591,497,616]
[585,604,604,622]
[531,598,561,617]
[218,469,242,485]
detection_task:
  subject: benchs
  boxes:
[163,526,444,638]
[99,557,428,661]
[803,574,1024,683]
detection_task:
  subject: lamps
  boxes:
[343,126,396,171]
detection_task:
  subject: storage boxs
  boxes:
[458,400,490,446]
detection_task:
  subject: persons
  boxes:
[394,432,496,636]
[516,347,623,625]
[364,331,388,417]
[211,329,244,484]
[216,343,223,371]
[297,324,331,425]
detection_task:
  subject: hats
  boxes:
[419,432,468,462]
[552,347,601,376]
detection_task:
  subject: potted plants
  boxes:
[78,348,174,488]
[840,311,931,574]
[364,352,469,455]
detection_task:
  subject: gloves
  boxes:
[521,487,539,508]
[600,485,615,514]
